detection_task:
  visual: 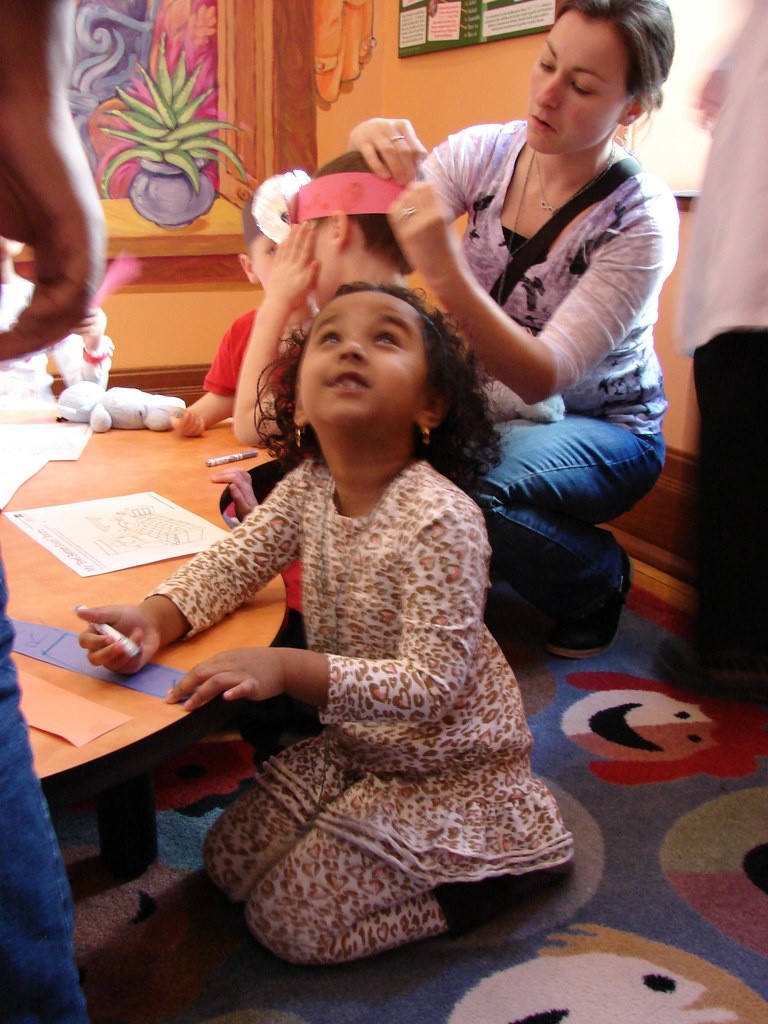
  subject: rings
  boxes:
[390,136,405,141]
[400,207,417,220]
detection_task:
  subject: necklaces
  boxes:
[498,143,616,306]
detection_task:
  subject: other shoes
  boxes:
[545,548,631,661]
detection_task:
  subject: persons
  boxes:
[351,0,680,655]
[662,0,768,706]
[0,1,115,1024]
[75,281,576,964]
[173,150,416,447]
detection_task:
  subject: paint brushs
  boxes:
[206,451,259,468]
[174,411,183,418]
[73,605,143,661]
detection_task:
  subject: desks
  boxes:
[0,417,305,881]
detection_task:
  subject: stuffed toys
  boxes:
[57,381,186,433]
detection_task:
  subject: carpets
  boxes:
[0,579,768,1024]
